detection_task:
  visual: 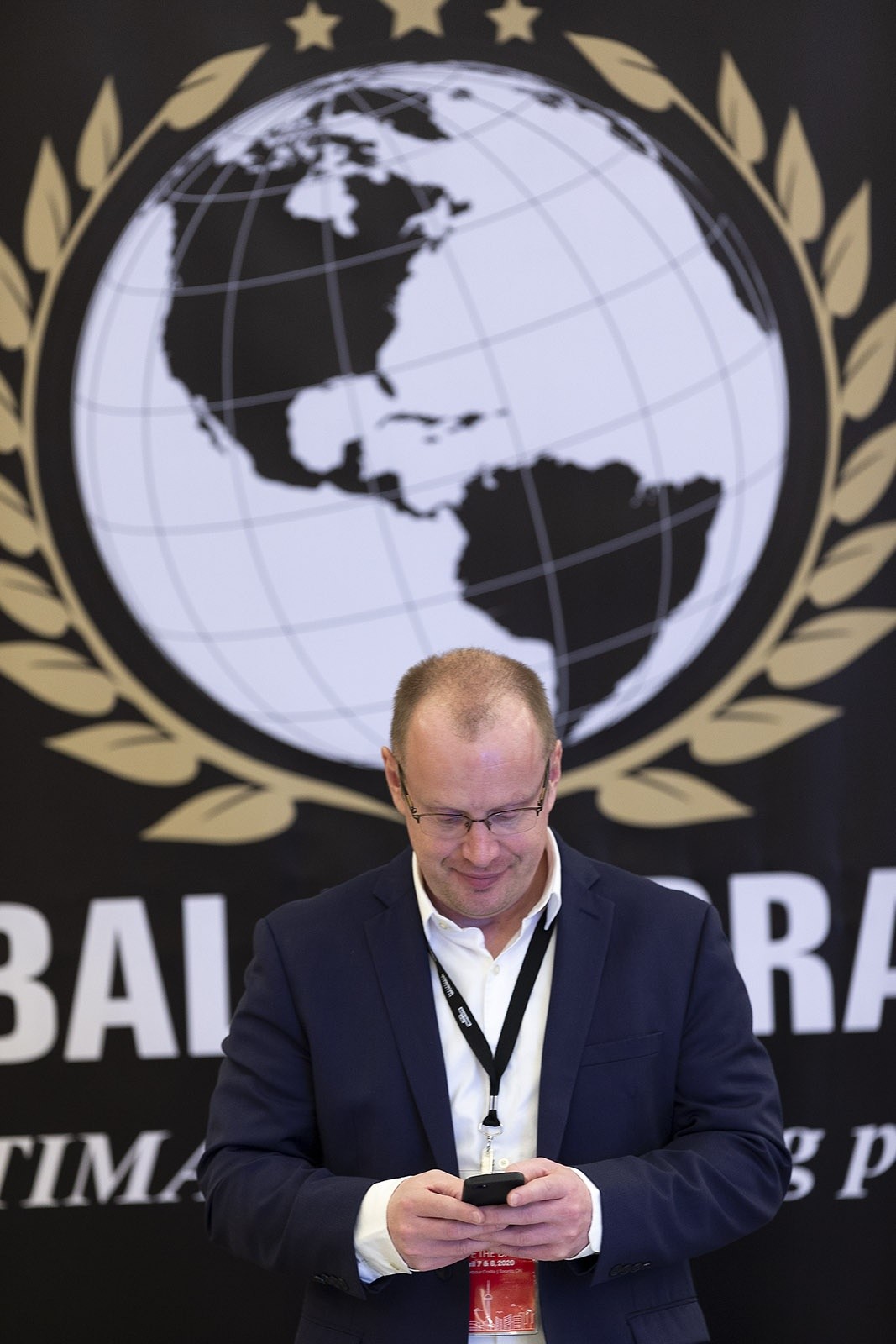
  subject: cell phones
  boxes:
[461,1171,525,1209]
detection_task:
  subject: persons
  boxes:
[200,645,794,1344]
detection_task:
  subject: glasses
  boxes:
[398,758,551,838]
